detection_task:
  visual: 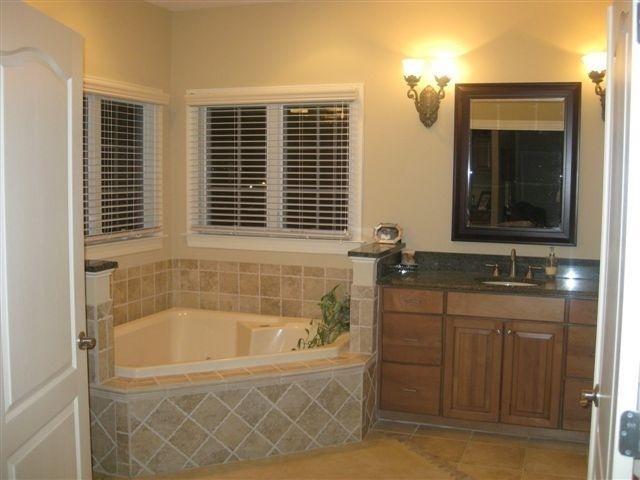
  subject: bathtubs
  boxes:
[113,306,350,378]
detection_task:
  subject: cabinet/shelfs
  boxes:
[375,288,597,446]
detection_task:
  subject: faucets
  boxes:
[509,248,517,277]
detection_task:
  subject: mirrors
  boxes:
[452,82,582,244]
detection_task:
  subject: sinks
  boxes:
[476,276,544,286]
